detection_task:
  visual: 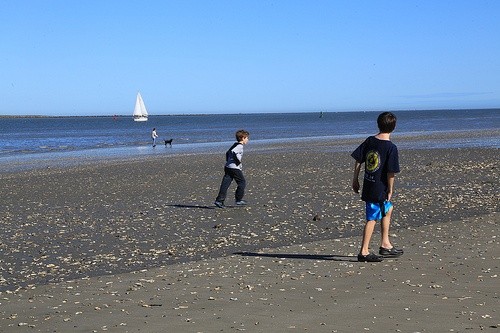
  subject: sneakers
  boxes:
[358,251,383,262]
[235,200,248,205]
[215,201,225,208]
[379,246,403,257]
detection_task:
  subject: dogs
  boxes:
[163,138,173,148]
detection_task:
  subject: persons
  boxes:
[215,129,248,208]
[151,128,158,147]
[351,113,403,263]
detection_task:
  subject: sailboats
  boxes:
[132,92,149,122]
